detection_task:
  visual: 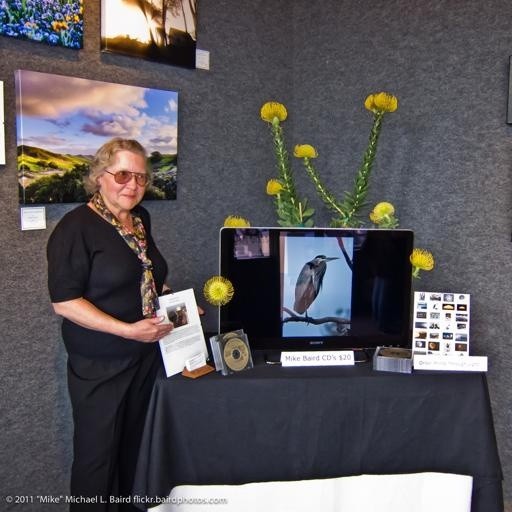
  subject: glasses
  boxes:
[101,167,150,187]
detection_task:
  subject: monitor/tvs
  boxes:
[218,225,413,364]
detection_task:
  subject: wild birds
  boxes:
[294,254,340,327]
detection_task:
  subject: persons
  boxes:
[47,136,205,512]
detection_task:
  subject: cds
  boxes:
[223,337,249,371]
[224,332,238,338]
[378,349,410,358]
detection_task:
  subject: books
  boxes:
[155,288,210,378]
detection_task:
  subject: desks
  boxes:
[132,333,503,511]
